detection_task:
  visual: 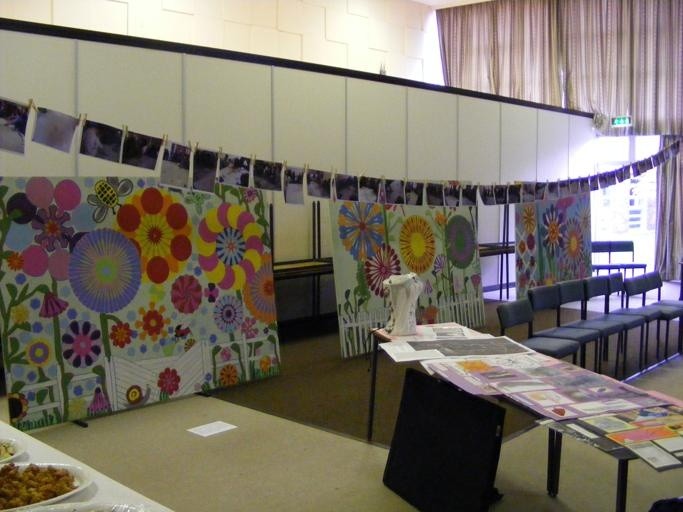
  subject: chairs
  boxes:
[494,239,683,379]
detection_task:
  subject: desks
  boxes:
[363,323,682,511]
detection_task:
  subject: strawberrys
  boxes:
[552,408,565,417]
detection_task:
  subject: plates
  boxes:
[1,435,93,512]
